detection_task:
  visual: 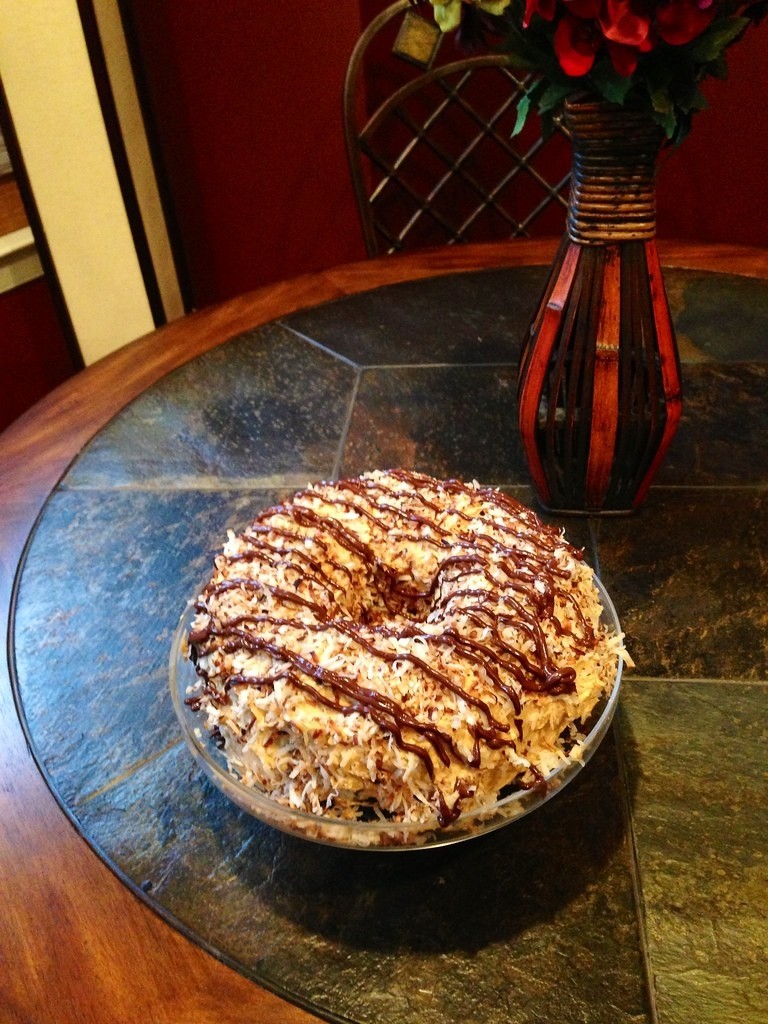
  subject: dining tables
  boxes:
[0,236,768,1024]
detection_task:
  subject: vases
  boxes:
[512,102,689,519]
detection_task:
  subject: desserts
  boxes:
[188,469,617,823]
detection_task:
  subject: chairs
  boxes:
[342,0,573,258]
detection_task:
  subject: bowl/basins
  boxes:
[171,521,623,856]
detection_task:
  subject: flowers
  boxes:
[498,0,761,124]
